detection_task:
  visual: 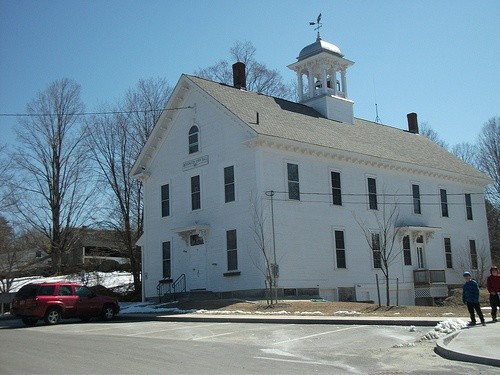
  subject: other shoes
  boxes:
[482,322,486,326]
[493,318,497,321]
[468,322,476,326]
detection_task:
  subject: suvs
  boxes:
[10,282,121,325]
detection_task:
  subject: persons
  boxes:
[487,265,500,322]
[460,271,487,326]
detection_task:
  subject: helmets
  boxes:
[463,272,470,276]
